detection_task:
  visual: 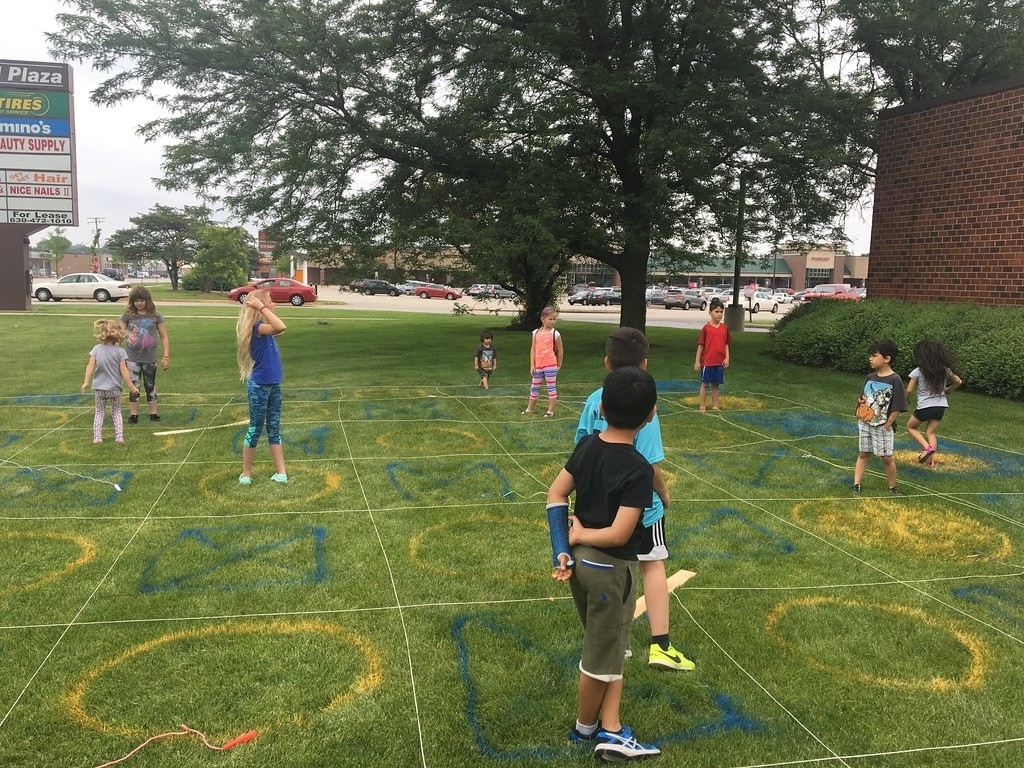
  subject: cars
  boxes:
[566,282,866,314]
[30,273,133,303]
[349,279,517,301]
[127,270,185,279]
[227,276,318,306]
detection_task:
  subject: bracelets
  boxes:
[163,356,169,358]
[260,306,268,314]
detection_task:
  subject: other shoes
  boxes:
[700,407,706,413]
[93,439,103,444]
[150,414,160,423]
[543,413,554,418]
[712,406,720,412]
[271,473,288,483]
[852,484,861,496]
[521,411,533,415]
[115,438,124,443]
[928,461,935,467]
[128,415,139,425]
[889,486,903,496]
[239,473,252,485]
[917,445,935,463]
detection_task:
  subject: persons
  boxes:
[521,307,563,417]
[235,290,288,485]
[575,327,695,670]
[81,319,139,444]
[853,339,909,496]
[115,286,169,423]
[473,331,497,389]
[694,300,732,412]
[546,366,661,764]
[906,339,962,467]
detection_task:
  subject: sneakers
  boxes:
[625,651,632,659]
[648,642,696,672]
[595,725,661,763]
[570,723,602,744]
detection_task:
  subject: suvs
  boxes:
[101,267,125,281]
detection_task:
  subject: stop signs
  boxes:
[744,285,755,298]
[93,257,99,271]
